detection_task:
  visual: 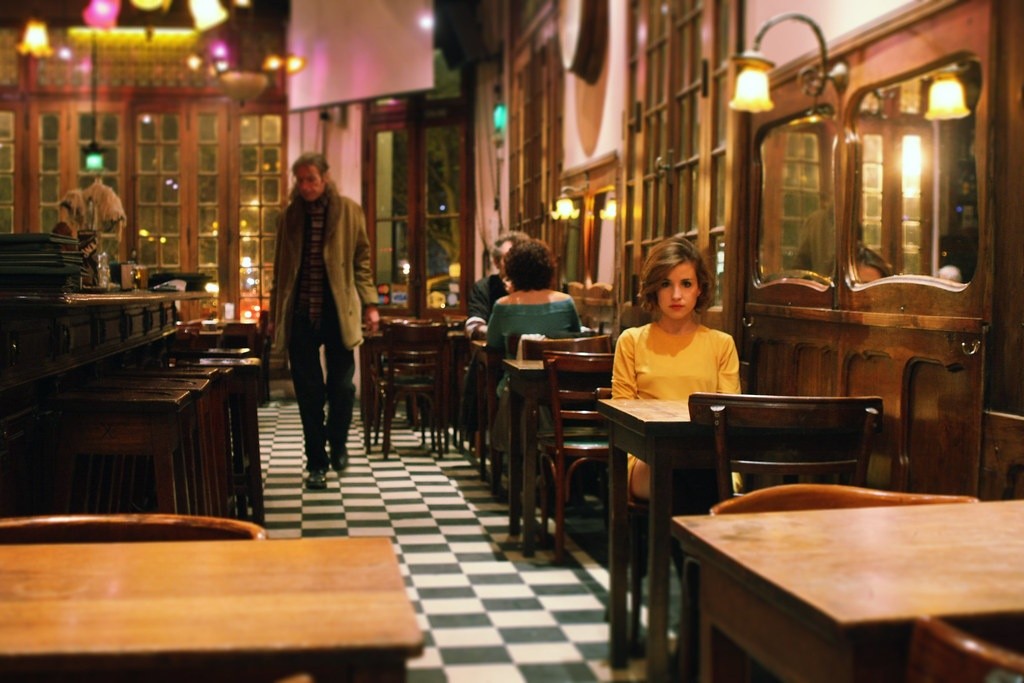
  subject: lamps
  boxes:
[15,0,307,109]
[727,13,850,114]
[599,198,617,220]
[872,65,973,122]
[550,172,590,219]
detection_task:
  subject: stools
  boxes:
[43,345,266,525]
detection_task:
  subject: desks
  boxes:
[0,535,427,683]
[502,359,752,558]
[360,331,465,457]
[447,331,467,456]
[669,499,1024,683]
[600,399,692,683]
[473,339,506,502]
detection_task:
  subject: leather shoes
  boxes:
[306,469,327,489]
[330,442,349,470]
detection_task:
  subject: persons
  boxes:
[611,239,742,503]
[266,152,379,488]
[791,191,975,284]
[52,189,87,252]
[464,230,584,503]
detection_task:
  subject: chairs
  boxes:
[520,333,615,521]
[374,318,450,460]
[669,392,885,683]
[605,501,650,660]
[0,514,268,545]
[906,615,1024,683]
[709,483,981,516]
[537,351,614,553]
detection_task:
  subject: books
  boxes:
[0,233,89,292]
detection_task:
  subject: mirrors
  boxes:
[742,0,1000,320]
[554,158,621,304]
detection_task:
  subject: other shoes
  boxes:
[562,480,584,511]
